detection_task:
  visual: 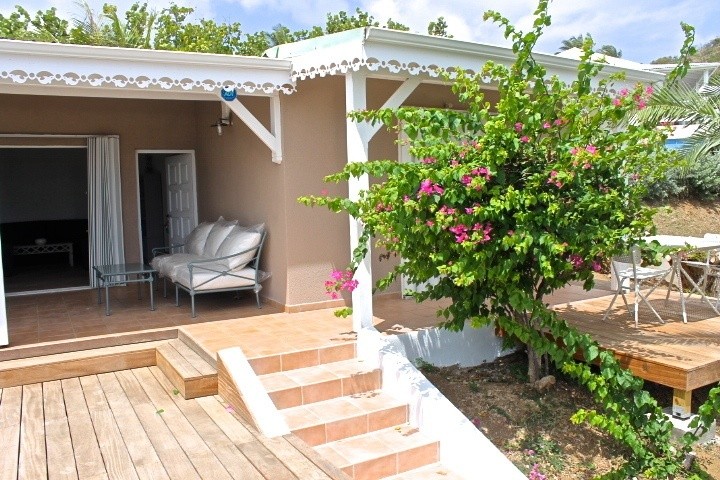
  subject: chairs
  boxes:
[600,234,720,327]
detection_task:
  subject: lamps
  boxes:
[211,111,233,137]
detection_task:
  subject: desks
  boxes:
[93,263,159,315]
[6,242,73,268]
[627,234,720,324]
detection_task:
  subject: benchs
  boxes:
[149,232,266,318]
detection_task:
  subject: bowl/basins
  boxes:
[34,238,46,245]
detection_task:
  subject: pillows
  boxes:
[185,216,264,272]
